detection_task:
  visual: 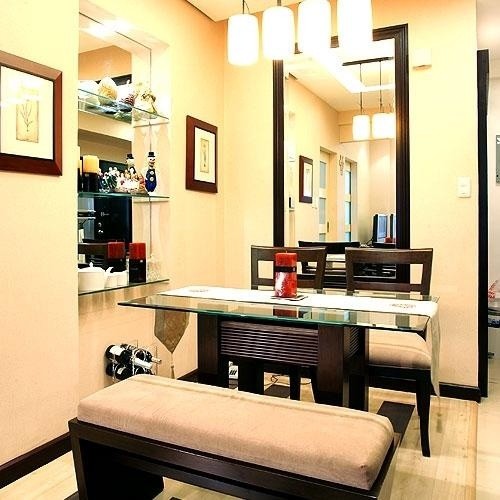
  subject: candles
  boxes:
[82,156,99,173]
[129,243,146,258]
[108,240,125,259]
[275,250,297,297]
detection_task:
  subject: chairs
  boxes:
[297,241,360,275]
[251,246,327,402]
[343,247,433,456]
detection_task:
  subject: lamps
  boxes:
[228,0,372,64]
[352,60,396,142]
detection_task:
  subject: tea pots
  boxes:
[77,262,114,292]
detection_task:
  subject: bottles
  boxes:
[105,344,162,380]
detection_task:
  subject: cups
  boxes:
[113,272,129,287]
[105,273,118,289]
[82,172,102,192]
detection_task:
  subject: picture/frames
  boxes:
[1,50,62,176]
[186,115,218,193]
[299,155,313,203]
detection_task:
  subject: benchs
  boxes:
[68,374,403,499]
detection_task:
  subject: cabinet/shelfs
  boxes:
[78,88,170,296]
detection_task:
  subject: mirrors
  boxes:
[79,10,152,272]
[272,23,410,283]
